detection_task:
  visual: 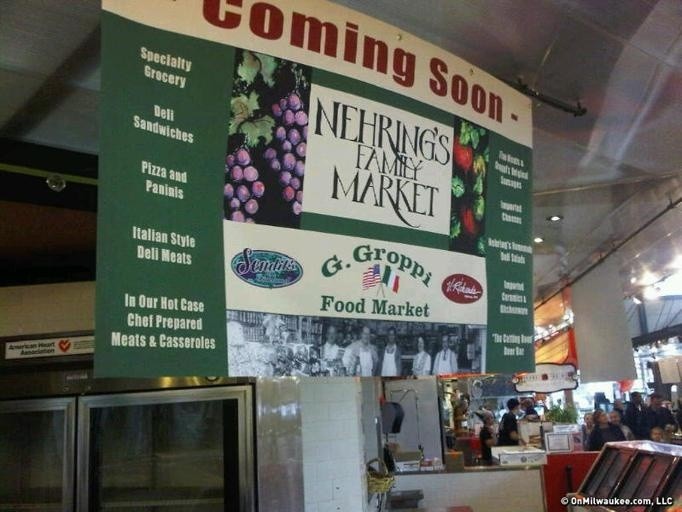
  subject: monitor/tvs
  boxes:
[0,395,77,512]
[76,383,258,512]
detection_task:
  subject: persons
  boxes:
[411,334,432,377]
[581,391,682,451]
[374,326,402,377]
[321,324,344,377]
[450,388,469,430]
[341,325,379,377]
[432,335,458,375]
[474,398,539,466]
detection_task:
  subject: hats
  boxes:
[507,398,522,408]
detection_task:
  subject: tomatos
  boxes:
[453,135,474,173]
[460,208,478,237]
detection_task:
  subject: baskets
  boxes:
[367,456,395,493]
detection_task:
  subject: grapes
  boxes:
[224,147,265,224]
[259,90,308,215]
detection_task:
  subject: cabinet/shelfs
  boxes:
[564,437,681,511]
